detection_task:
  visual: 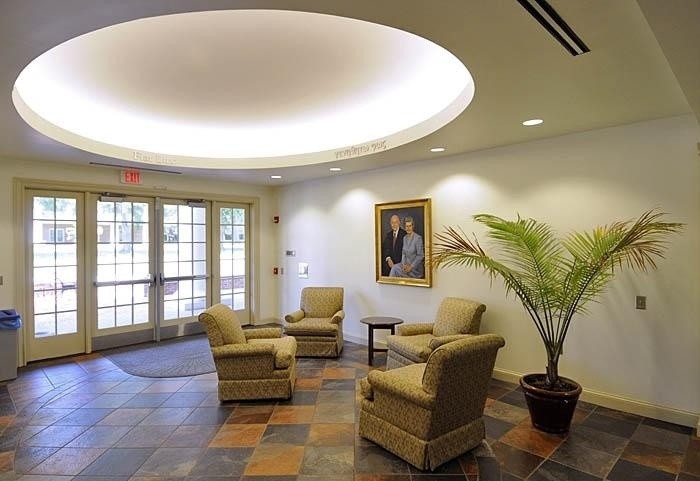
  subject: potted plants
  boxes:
[424,210,688,438]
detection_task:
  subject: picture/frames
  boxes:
[374,198,434,289]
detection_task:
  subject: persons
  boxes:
[389,216,424,278]
[382,215,408,276]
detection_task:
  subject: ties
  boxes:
[392,233,396,253]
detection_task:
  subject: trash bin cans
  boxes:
[0,309,23,382]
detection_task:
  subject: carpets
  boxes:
[100,333,218,379]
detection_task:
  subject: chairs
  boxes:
[197,303,298,405]
[283,286,346,361]
[358,297,507,472]
